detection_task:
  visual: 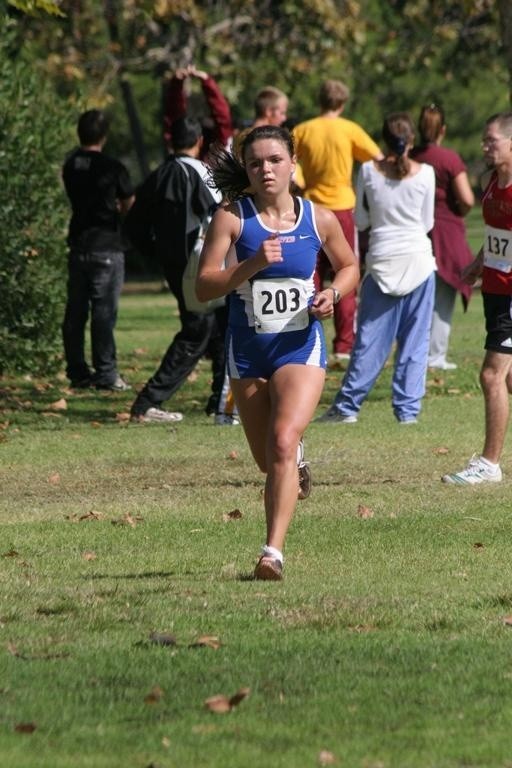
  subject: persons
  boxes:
[126,112,245,429]
[307,108,436,426]
[231,84,305,204]
[194,123,365,581]
[61,107,137,393]
[159,63,237,169]
[436,113,512,486]
[287,76,386,360]
[405,102,480,372]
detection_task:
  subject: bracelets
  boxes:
[328,285,342,306]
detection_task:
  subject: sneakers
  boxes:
[297,437,311,500]
[399,416,419,424]
[130,407,183,423]
[254,552,283,579]
[312,410,357,423]
[442,456,501,484]
[110,373,130,391]
[428,357,457,370]
[216,415,239,425]
